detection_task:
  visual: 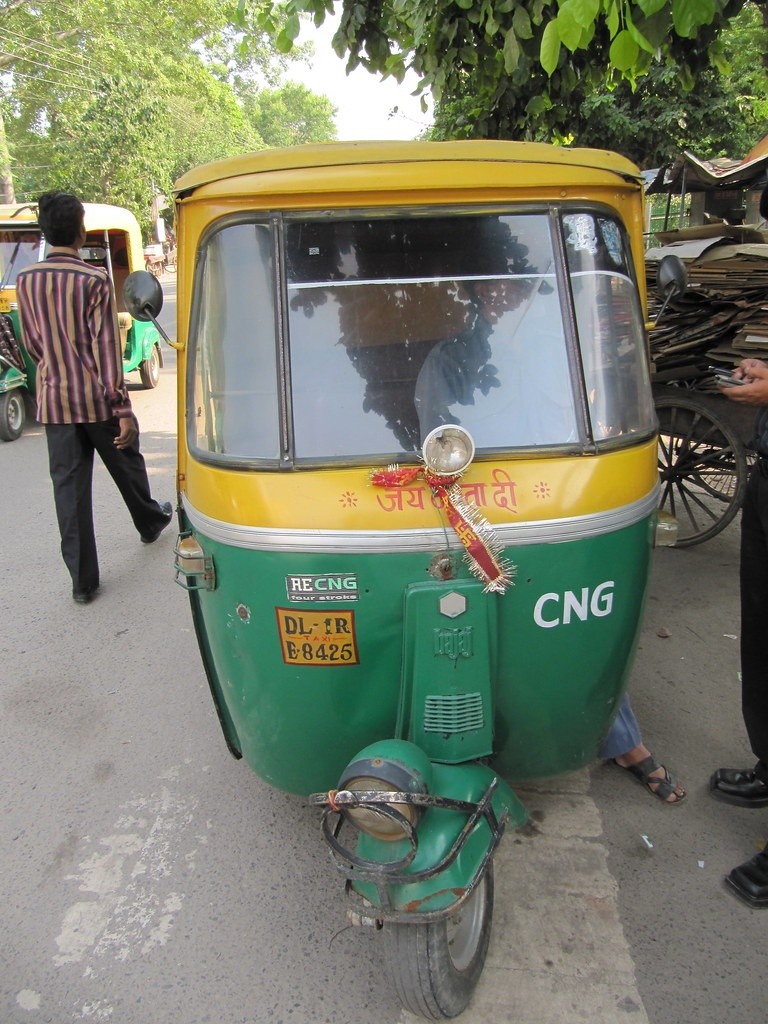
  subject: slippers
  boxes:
[611,754,687,805]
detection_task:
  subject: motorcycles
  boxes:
[0,202,163,442]
[121,140,692,1020]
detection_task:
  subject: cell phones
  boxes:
[709,366,735,377]
[715,374,746,389]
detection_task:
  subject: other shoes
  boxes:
[73,582,97,603]
[141,500,173,543]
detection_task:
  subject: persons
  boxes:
[417,237,686,805]
[707,190,768,910]
[2,231,40,285]
[15,193,173,603]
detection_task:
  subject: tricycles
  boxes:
[145,238,178,279]
[596,384,763,551]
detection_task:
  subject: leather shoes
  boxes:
[725,847,768,908]
[708,768,768,807]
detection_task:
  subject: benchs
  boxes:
[309,278,476,453]
[104,265,133,358]
